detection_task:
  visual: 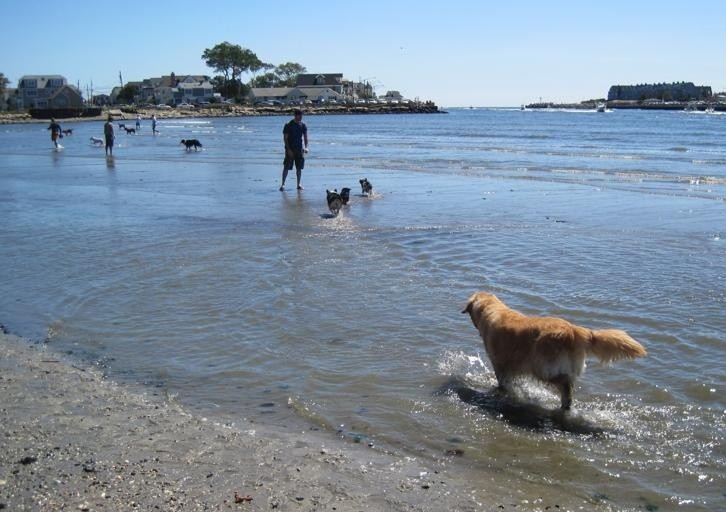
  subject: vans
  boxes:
[199,101,212,109]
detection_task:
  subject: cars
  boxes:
[156,104,171,110]
[176,102,195,110]
[254,98,409,107]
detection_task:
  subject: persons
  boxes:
[104,117,115,157]
[134,114,141,130]
[150,114,159,136]
[278,110,309,192]
[46,117,64,150]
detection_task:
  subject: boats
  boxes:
[520,104,525,110]
[597,102,606,112]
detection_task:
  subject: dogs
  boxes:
[117,123,135,135]
[359,178,373,196]
[325,188,344,214]
[460,290,649,411]
[61,128,74,136]
[340,187,352,205]
[90,136,103,145]
[180,139,202,152]
